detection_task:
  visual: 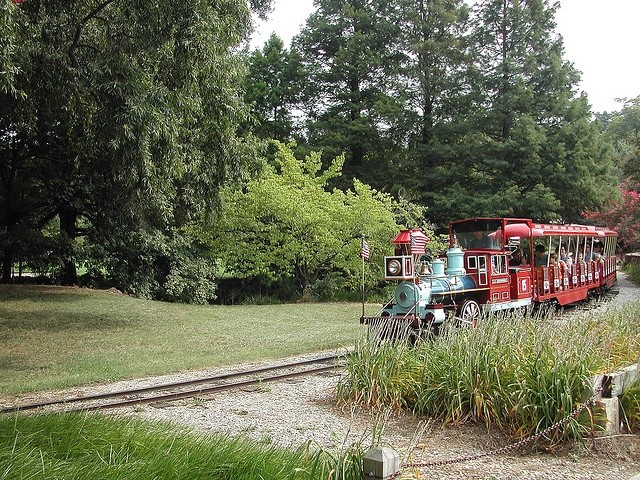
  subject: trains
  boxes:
[356,218,618,348]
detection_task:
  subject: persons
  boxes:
[534,244,548,265]
[518,252,530,267]
[468,231,493,248]
[549,240,605,281]
[509,249,521,266]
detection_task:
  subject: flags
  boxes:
[360,239,370,258]
[410,234,427,254]
